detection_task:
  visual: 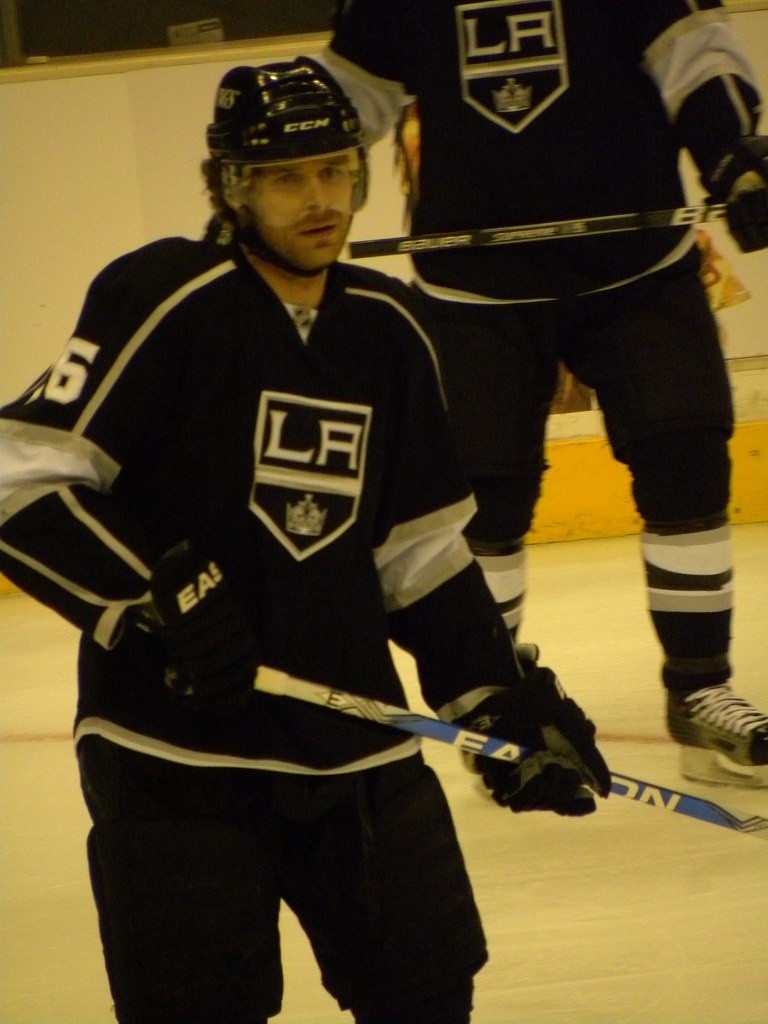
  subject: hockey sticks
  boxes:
[329,195,730,258]
[248,664,767,835]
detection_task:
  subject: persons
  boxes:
[201,0,768,792]
[3,49,612,1021]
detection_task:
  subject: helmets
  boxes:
[209,54,365,167]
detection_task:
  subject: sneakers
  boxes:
[667,683,768,789]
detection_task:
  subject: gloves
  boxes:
[696,143,768,251]
[123,545,329,732]
[439,642,610,816]
